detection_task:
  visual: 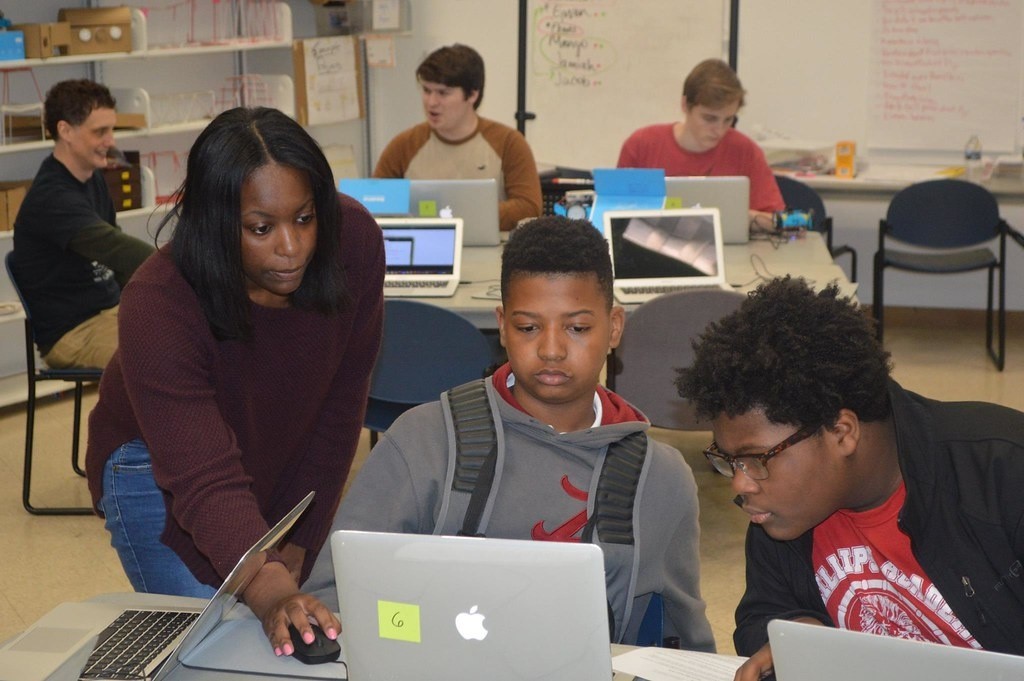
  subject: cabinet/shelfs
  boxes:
[0,0,294,407]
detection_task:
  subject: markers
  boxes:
[552,178,593,185]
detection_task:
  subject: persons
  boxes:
[374,43,542,231]
[13,78,159,368]
[616,58,786,236]
[669,273,1024,681]
[301,215,716,653]
[85,106,385,656]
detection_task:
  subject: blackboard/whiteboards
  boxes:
[517,0,1024,182]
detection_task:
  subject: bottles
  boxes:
[965,133,983,185]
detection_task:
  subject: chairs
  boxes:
[773,173,858,285]
[358,299,498,455]
[604,288,751,431]
[3,250,107,517]
[873,179,1006,372]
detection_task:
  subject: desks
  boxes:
[386,231,862,331]
[0,589,752,681]
[769,162,1024,206]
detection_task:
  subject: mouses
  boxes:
[288,622,341,666]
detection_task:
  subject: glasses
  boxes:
[703,414,829,480]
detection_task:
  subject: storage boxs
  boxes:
[6,21,71,60]
[100,151,142,215]
[57,7,131,55]
[0,30,27,61]
[0,177,36,232]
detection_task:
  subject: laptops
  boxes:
[604,173,751,305]
[370,179,504,297]
[331,529,614,681]
[0,490,316,681]
[767,620,1024,681]
